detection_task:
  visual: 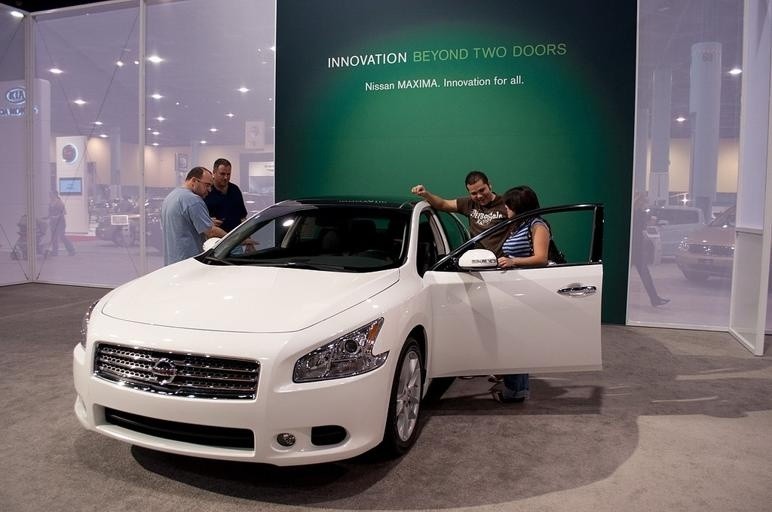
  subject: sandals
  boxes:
[491,389,528,404]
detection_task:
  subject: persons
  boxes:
[411,171,514,258]
[49,189,77,257]
[631,190,671,307]
[492,186,552,404]
[160,167,260,266]
[203,158,248,255]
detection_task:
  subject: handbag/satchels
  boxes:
[528,217,567,265]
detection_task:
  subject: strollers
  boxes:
[11,215,50,261]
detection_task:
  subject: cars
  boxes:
[72,196,604,467]
[96,198,163,247]
[643,205,735,281]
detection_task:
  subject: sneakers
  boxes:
[488,375,504,382]
